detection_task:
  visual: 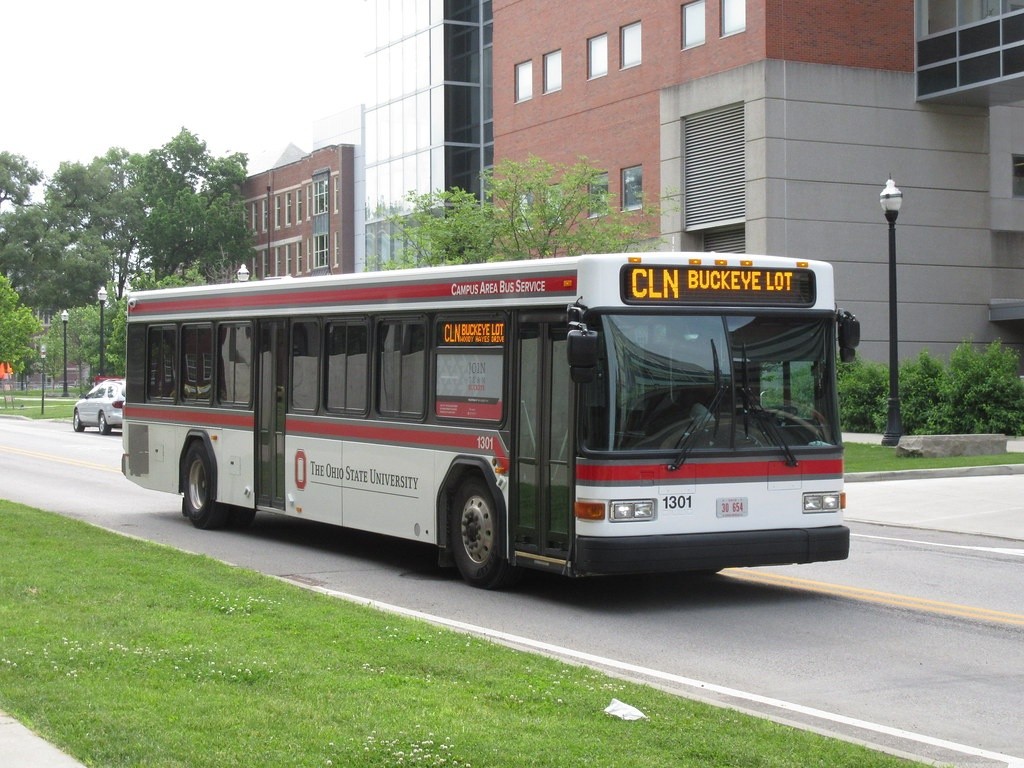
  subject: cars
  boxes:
[73,378,125,435]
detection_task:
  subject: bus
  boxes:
[120,252,860,593]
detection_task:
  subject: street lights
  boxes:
[61,310,70,398]
[881,175,904,448]
[97,285,107,377]
